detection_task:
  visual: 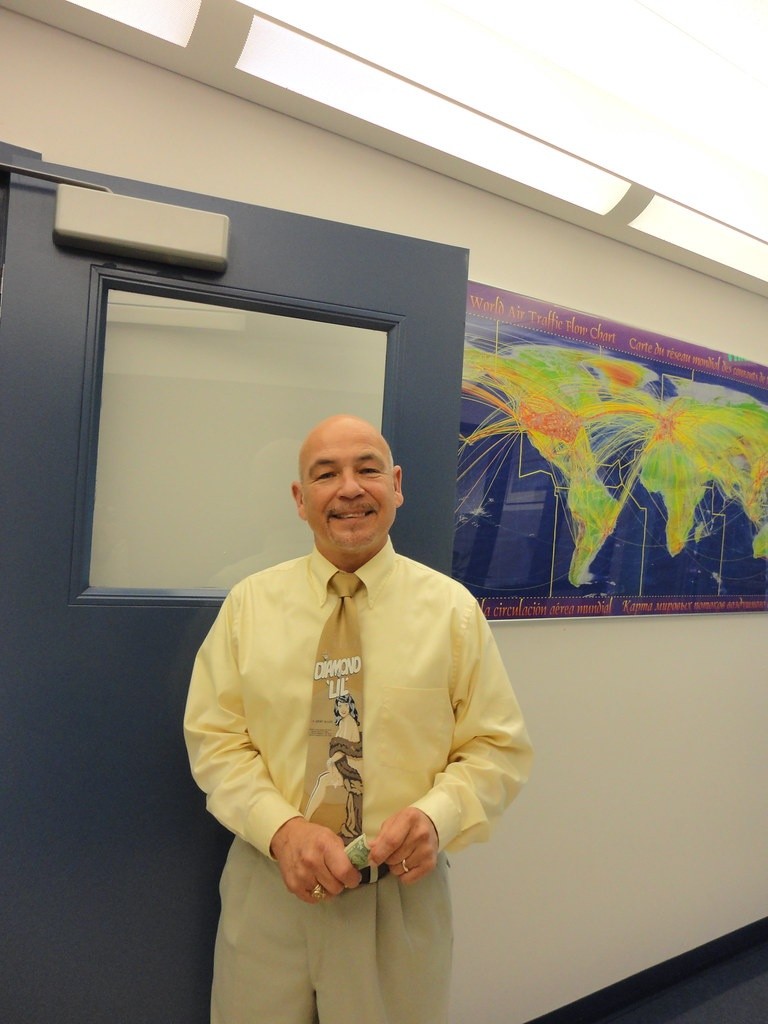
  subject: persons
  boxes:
[182,415,537,1024]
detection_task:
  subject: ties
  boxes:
[298,570,365,847]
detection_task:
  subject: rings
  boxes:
[310,883,327,902]
[403,859,409,872]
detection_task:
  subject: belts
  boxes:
[353,861,394,883]
